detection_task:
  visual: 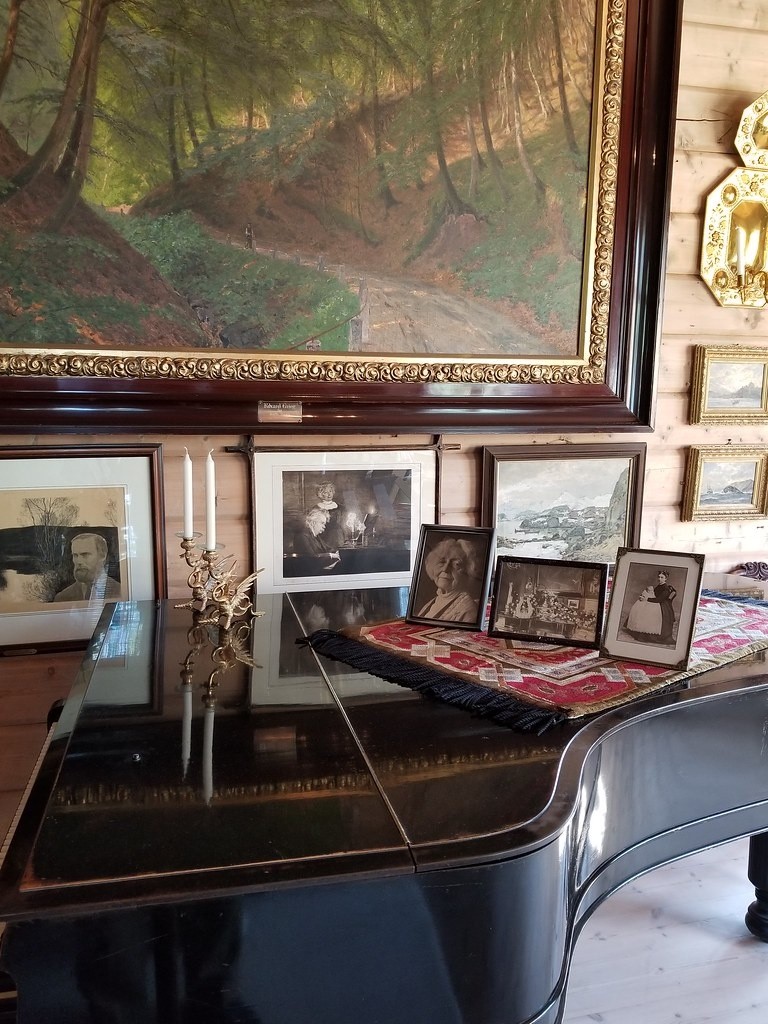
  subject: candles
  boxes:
[182,686,192,778]
[203,712,214,806]
[205,449,215,551]
[183,447,194,541]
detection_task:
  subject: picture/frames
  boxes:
[681,445,768,523]
[481,442,647,576]
[247,596,425,715]
[689,344,768,426]
[487,555,608,650]
[225,435,460,595]
[79,599,166,720]
[0,0,684,435]
[46,704,566,815]
[0,442,168,657]
[405,524,497,632]
[599,547,705,672]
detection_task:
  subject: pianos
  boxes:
[322,543,410,574]
[2,585,767,1023]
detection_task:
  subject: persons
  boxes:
[56,534,123,603]
[627,569,676,645]
[296,507,343,568]
[418,539,476,623]
[311,479,340,548]
